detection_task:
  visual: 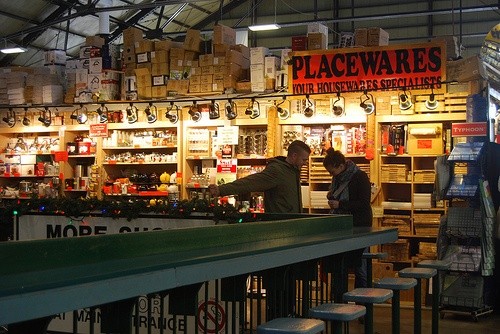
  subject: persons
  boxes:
[208,140,311,318]
[323,146,373,324]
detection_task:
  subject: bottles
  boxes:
[190,163,209,203]
[102,130,177,147]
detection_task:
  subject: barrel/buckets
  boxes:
[20,182,30,191]
[66,142,96,155]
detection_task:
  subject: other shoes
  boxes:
[359,316,367,323]
[286,312,300,318]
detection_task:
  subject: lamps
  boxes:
[2,85,439,129]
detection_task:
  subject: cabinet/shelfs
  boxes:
[277,114,373,305]
[183,117,276,214]
[435,122,500,322]
[99,121,181,214]
[0,126,59,204]
[375,113,466,310]
[60,124,98,203]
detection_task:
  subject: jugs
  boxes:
[129,171,178,193]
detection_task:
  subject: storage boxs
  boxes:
[432,36,486,82]
[0,25,251,104]
[293,24,389,50]
[250,46,292,92]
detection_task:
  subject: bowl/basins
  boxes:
[64,191,87,200]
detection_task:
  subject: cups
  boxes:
[65,165,92,190]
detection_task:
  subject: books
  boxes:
[310,157,448,262]
[447,142,484,161]
[441,178,497,308]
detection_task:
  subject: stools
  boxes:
[343,288,394,334]
[417,260,452,334]
[373,277,418,334]
[259,318,326,334]
[399,268,437,334]
[309,303,366,334]
